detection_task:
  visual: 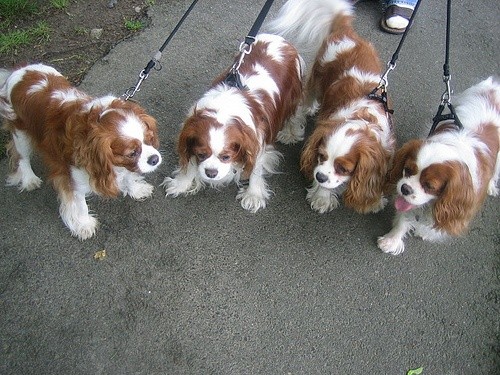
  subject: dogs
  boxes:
[263,0,396,214]
[0,61,163,241]
[377,75,500,256]
[158,32,307,214]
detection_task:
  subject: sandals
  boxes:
[380,4,414,35]
